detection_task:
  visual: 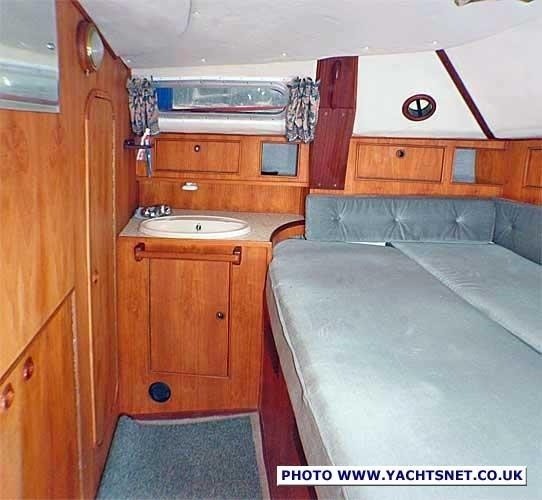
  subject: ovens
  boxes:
[267,193,541,500]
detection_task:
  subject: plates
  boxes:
[115,236,272,420]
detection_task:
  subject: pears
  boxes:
[134,127,152,177]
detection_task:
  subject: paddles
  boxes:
[94,412,271,500]
